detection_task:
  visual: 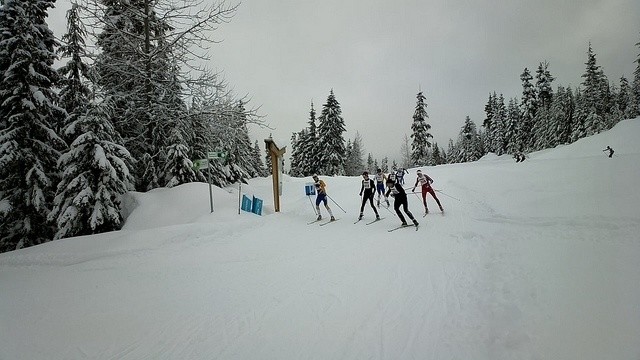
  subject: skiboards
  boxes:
[354,217,385,225]
[308,217,341,226]
[423,211,444,217]
[378,205,389,209]
[388,224,418,232]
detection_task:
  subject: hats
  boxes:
[387,179,395,183]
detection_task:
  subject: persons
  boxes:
[312,174,335,221]
[602,146,614,158]
[358,172,380,220]
[512,153,526,163]
[388,167,409,184]
[411,170,444,211]
[373,169,388,206]
[385,179,419,226]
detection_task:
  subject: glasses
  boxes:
[313,176,317,178]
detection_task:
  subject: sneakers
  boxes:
[414,221,419,226]
[402,222,407,225]
[377,216,381,219]
[318,216,322,220]
[359,217,363,220]
[331,216,335,221]
